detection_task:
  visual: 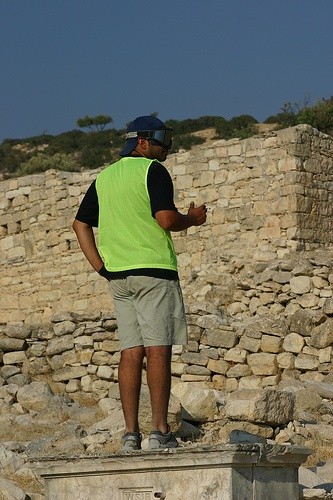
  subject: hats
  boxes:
[115,113,174,160]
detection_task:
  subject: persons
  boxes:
[72,116,207,450]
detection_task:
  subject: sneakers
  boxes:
[117,425,182,453]
